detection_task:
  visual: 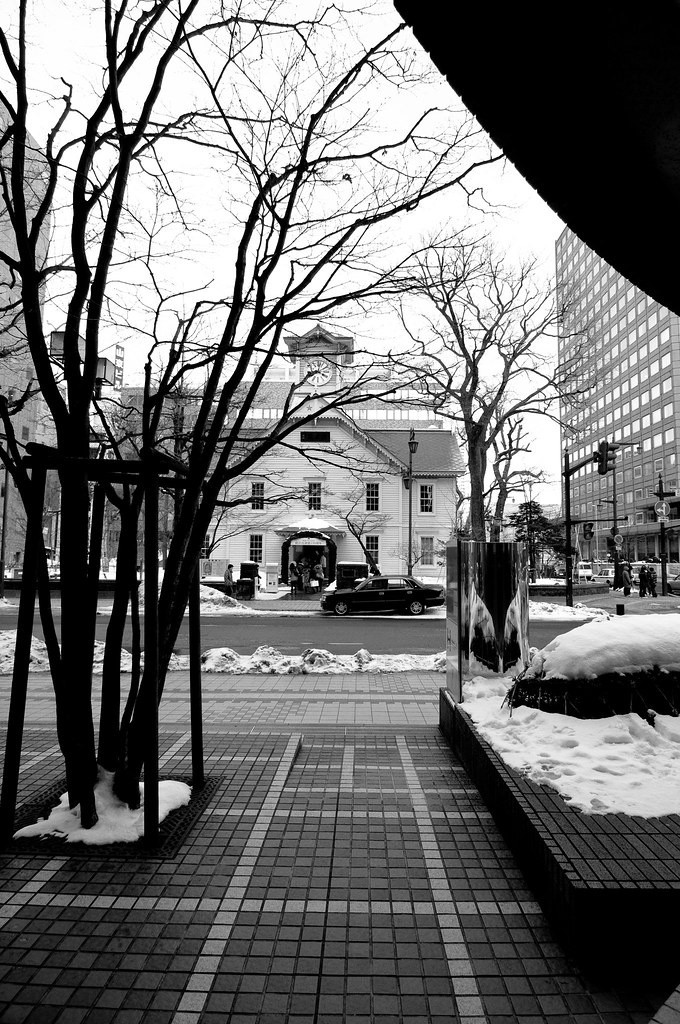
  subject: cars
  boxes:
[668,573,680,593]
[591,569,634,588]
[320,574,446,616]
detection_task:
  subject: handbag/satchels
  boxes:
[310,580,319,587]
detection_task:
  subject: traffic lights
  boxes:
[598,441,620,475]
[583,522,594,540]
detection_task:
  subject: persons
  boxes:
[622,565,633,597]
[290,550,328,595]
[224,564,234,596]
[639,565,657,597]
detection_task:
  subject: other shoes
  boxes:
[319,590,321,592]
[290,593,293,595]
[294,592,296,595]
[317,590,318,592]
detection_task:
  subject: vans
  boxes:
[576,562,592,581]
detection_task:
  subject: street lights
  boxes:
[407,427,420,574]
[597,499,621,591]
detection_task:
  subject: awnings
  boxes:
[274,526,346,537]
